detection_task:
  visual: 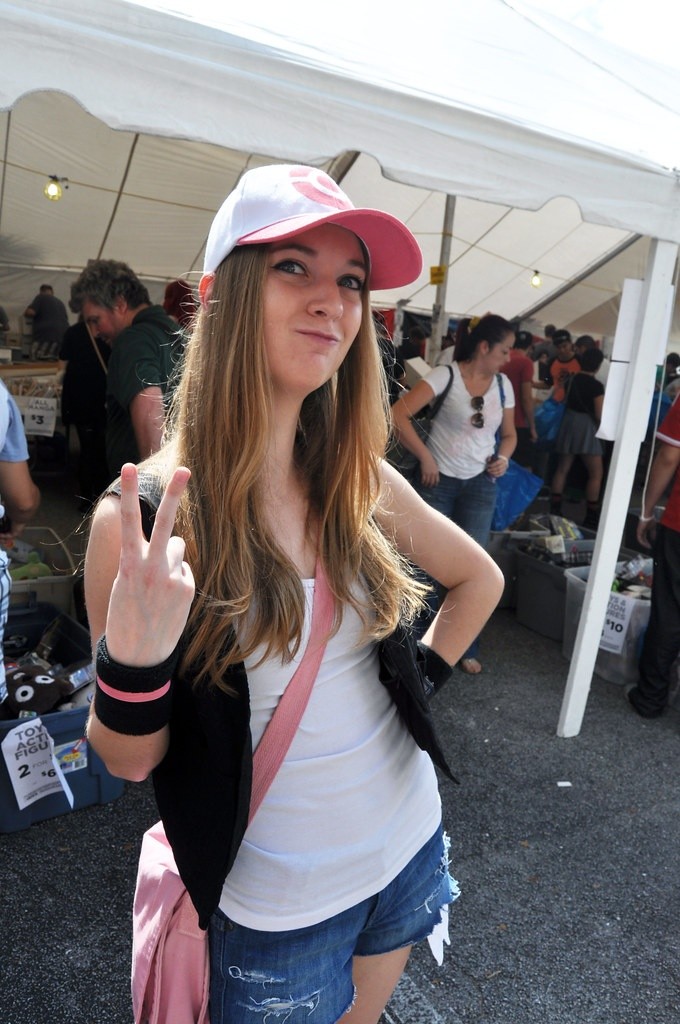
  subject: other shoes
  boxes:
[536,487,550,500]
[570,491,580,502]
[625,681,639,702]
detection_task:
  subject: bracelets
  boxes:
[94,635,172,736]
[416,640,453,702]
[498,456,509,469]
[640,516,655,522]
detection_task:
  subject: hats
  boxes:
[552,329,572,347]
[515,330,534,352]
[202,164,423,291]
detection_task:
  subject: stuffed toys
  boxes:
[4,660,75,716]
[8,540,65,579]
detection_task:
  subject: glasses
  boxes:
[470,396,485,429]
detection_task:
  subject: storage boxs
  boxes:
[9,527,77,615]
[0,315,33,355]
[0,601,125,834]
[486,514,654,686]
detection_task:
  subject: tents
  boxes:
[0,0,680,736]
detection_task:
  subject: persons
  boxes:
[25,283,69,360]
[646,351,680,439]
[625,390,680,719]
[68,258,189,537]
[390,313,517,672]
[500,325,614,525]
[0,306,9,331]
[376,322,455,480]
[0,383,40,703]
[83,162,505,1024]
[58,322,112,512]
[163,279,197,326]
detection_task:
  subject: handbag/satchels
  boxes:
[131,818,212,1024]
[531,397,567,451]
[490,453,544,531]
[385,364,454,470]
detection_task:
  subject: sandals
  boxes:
[459,653,482,675]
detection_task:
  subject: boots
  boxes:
[550,493,564,517]
[583,500,599,530]
